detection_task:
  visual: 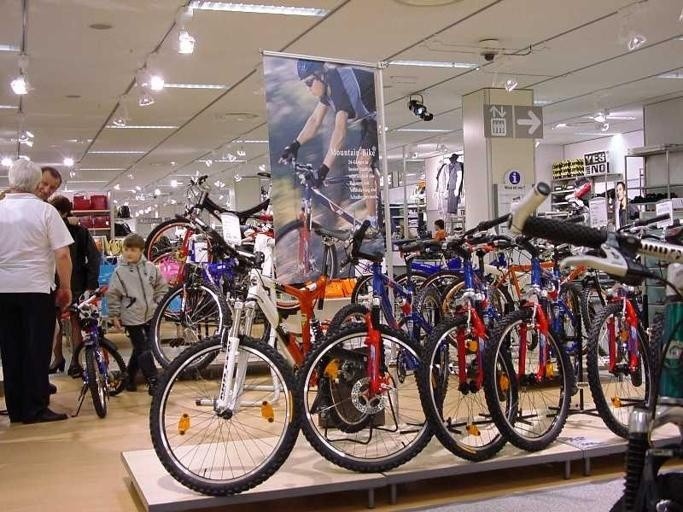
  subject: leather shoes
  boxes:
[10,409,67,423]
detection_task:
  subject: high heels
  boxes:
[68,361,81,377]
[48,359,64,373]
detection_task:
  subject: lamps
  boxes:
[628,33,646,51]
[406,92,434,122]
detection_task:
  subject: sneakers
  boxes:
[145,376,158,395]
[126,373,137,391]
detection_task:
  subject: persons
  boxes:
[0,159,75,424]
[616,181,640,232]
[106,233,169,396]
[1,167,62,199]
[278,60,379,235]
[48,195,101,376]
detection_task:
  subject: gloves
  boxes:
[279,140,300,166]
[296,164,328,190]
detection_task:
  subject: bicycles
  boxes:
[273,154,387,313]
[58,285,129,418]
[503,180,683,512]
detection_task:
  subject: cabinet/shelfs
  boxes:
[550,177,577,219]
[382,205,424,228]
[71,189,115,240]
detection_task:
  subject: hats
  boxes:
[297,59,324,81]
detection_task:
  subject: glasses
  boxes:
[305,80,314,87]
